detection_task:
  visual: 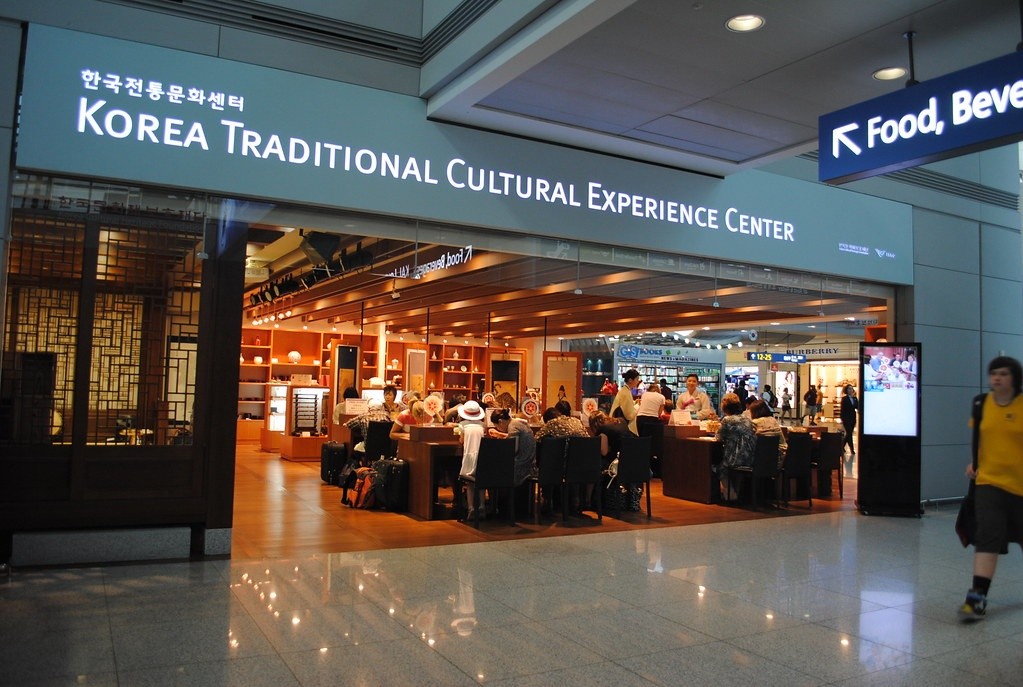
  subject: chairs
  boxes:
[353,421,396,469]
[456,432,844,527]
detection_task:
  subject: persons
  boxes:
[608,369,640,424]
[715,392,789,499]
[759,385,774,416]
[676,374,711,420]
[840,383,859,455]
[864,350,917,389]
[443,392,645,527]
[638,380,645,389]
[800,386,817,425]
[815,384,823,420]
[636,383,665,477]
[660,379,673,412]
[332,385,445,459]
[603,378,613,394]
[781,388,793,423]
[733,380,748,402]
[953,356,1023,623]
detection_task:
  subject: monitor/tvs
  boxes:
[863,345,917,437]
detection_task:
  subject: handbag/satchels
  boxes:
[955,479,975,549]
[608,451,619,477]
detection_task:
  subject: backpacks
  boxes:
[338,451,373,489]
[765,391,778,408]
[342,466,378,509]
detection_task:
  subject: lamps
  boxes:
[250,229,370,307]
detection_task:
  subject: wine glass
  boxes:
[451,366,455,372]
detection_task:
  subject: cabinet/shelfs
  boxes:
[236,327,379,445]
[384,341,528,414]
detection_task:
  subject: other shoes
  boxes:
[459,507,476,521]
[957,589,986,619]
[478,505,486,520]
[842,447,846,452]
[851,450,856,455]
[810,422,817,425]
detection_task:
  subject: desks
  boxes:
[331,413,828,521]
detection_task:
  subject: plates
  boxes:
[461,366,467,372]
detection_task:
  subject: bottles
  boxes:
[239,353,244,365]
[453,349,459,359]
[325,359,330,367]
[255,336,260,346]
[430,379,435,389]
[288,351,301,363]
[431,351,437,360]
[391,358,399,369]
[327,342,331,348]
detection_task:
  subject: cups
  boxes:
[254,356,263,364]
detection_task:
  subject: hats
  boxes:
[458,400,485,420]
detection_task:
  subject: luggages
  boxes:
[371,456,410,513]
[321,440,346,485]
